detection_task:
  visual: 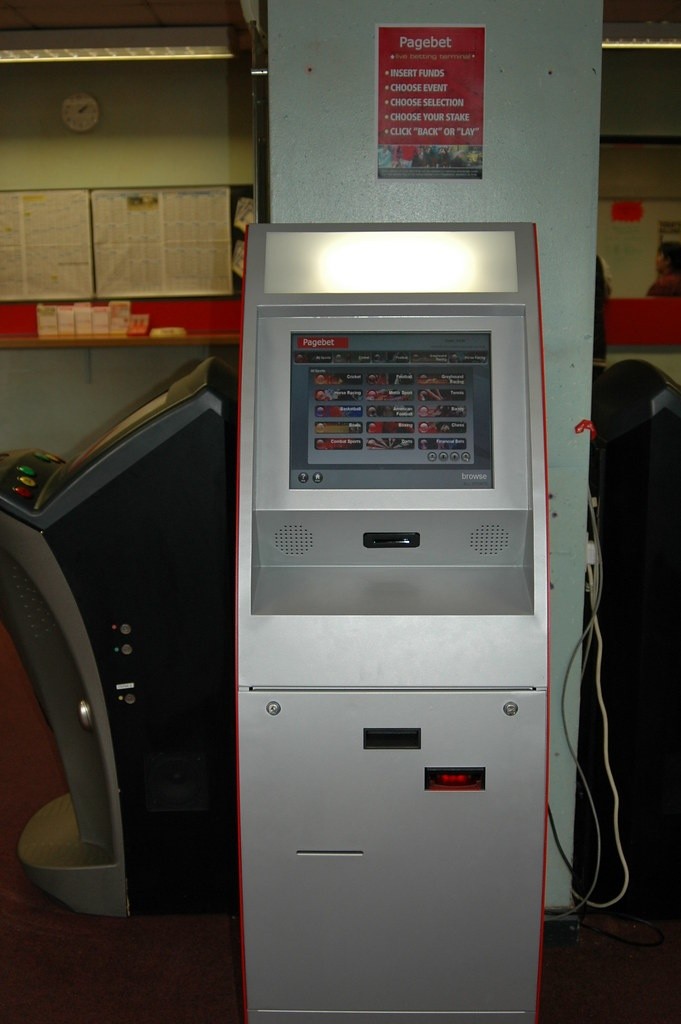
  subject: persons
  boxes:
[647,241,681,297]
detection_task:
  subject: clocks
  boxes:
[61,88,101,135]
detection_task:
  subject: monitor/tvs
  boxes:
[62,385,172,479]
